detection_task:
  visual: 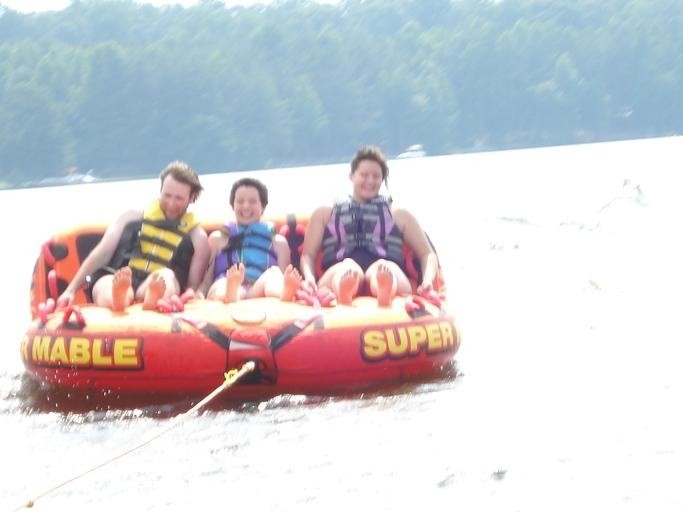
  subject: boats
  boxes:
[19,218,462,401]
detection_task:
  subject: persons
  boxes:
[51,160,211,314]
[188,175,304,307]
[299,143,439,309]
